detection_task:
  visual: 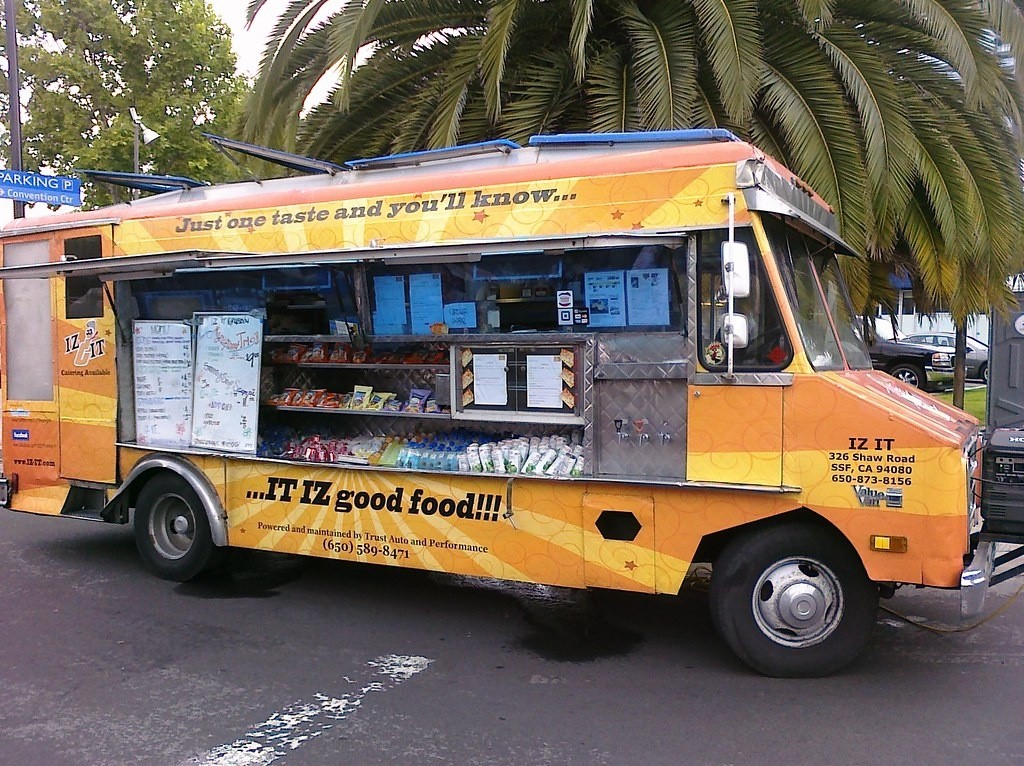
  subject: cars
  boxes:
[855,315,907,344]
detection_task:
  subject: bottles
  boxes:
[257,425,297,457]
[368,432,435,465]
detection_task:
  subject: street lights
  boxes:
[129,107,161,200]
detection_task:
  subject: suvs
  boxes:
[850,318,968,391]
[906,332,989,385]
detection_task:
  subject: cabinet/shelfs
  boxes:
[257,332,594,477]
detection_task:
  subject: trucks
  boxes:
[0,129,1024,679]
